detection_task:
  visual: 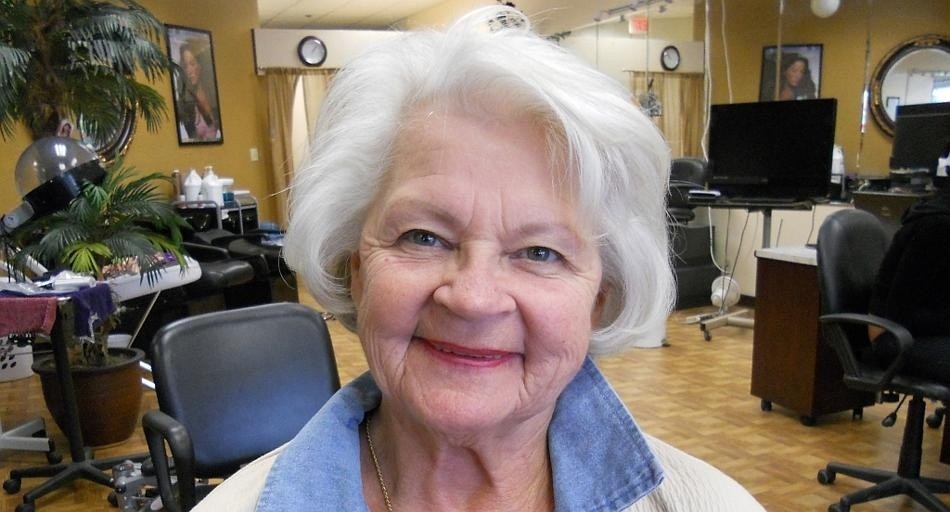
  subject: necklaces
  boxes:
[365,415,394,512]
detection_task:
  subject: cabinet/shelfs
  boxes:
[749,245,875,427]
[171,194,260,234]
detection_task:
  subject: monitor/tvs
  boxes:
[888,101,950,194]
[705,97,838,199]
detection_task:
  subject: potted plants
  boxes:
[12,148,193,446]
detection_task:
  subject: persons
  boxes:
[771,52,816,101]
[177,44,220,142]
[189,4,767,512]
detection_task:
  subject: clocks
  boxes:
[298,36,327,67]
[661,45,680,71]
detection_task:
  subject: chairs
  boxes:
[142,302,341,512]
[816,209,950,512]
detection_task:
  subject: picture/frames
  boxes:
[164,22,224,146]
[758,43,823,102]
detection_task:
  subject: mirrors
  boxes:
[868,33,950,138]
